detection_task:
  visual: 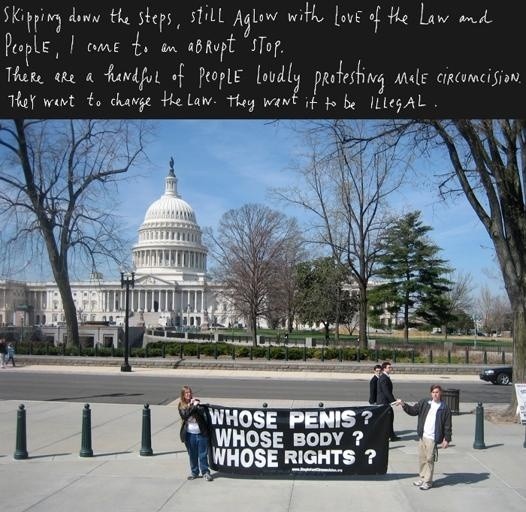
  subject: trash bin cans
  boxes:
[442,389,460,414]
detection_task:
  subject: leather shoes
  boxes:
[391,437,401,441]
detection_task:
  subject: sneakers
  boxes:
[413,480,422,486]
[203,472,212,481]
[188,475,197,480]
[420,482,432,490]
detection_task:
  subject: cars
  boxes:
[480,367,512,385]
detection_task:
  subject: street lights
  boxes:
[120,271,135,371]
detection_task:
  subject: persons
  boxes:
[5,341,17,367]
[368,364,382,406]
[376,362,402,441]
[397,384,452,490]
[178,384,214,482]
[0,338,8,368]
